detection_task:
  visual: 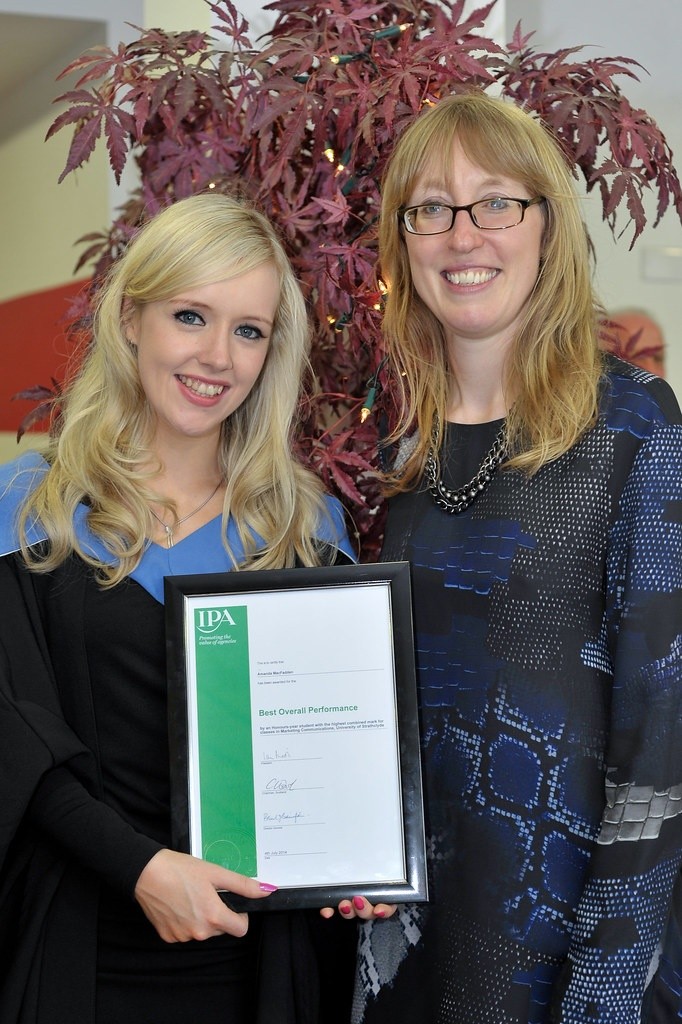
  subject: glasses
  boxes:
[397,196,545,235]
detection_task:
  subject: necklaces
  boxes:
[425,399,518,512]
[147,470,227,576]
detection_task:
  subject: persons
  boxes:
[333,93,682,1024]
[0,194,399,1024]
